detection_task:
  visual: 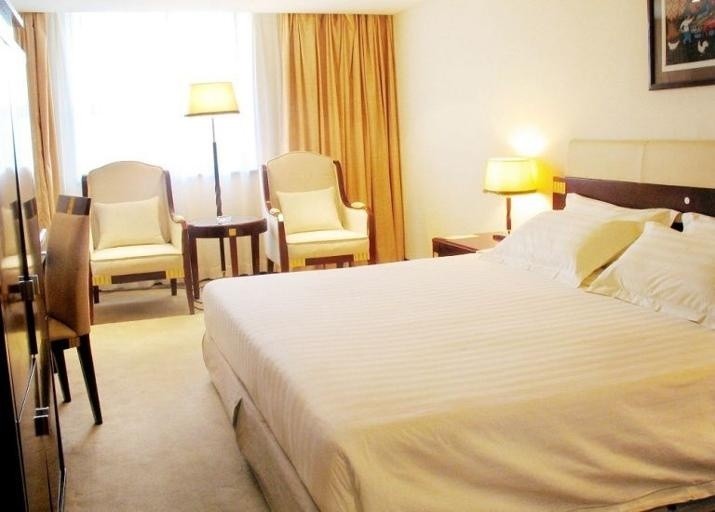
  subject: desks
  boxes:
[187,214,268,299]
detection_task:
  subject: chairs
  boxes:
[259,150,376,275]
[46,194,102,425]
[81,160,194,325]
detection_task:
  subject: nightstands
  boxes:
[432,232,506,257]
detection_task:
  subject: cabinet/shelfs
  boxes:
[0,0,66,512]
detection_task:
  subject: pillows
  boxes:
[477,192,715,330]
[275,187,342,235]
[94,196,165,250]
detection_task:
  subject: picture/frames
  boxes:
[646,0,715,91]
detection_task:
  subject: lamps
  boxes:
[482,157,536,241]
[185,82,242,279]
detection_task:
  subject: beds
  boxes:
[202,139,715,512]
